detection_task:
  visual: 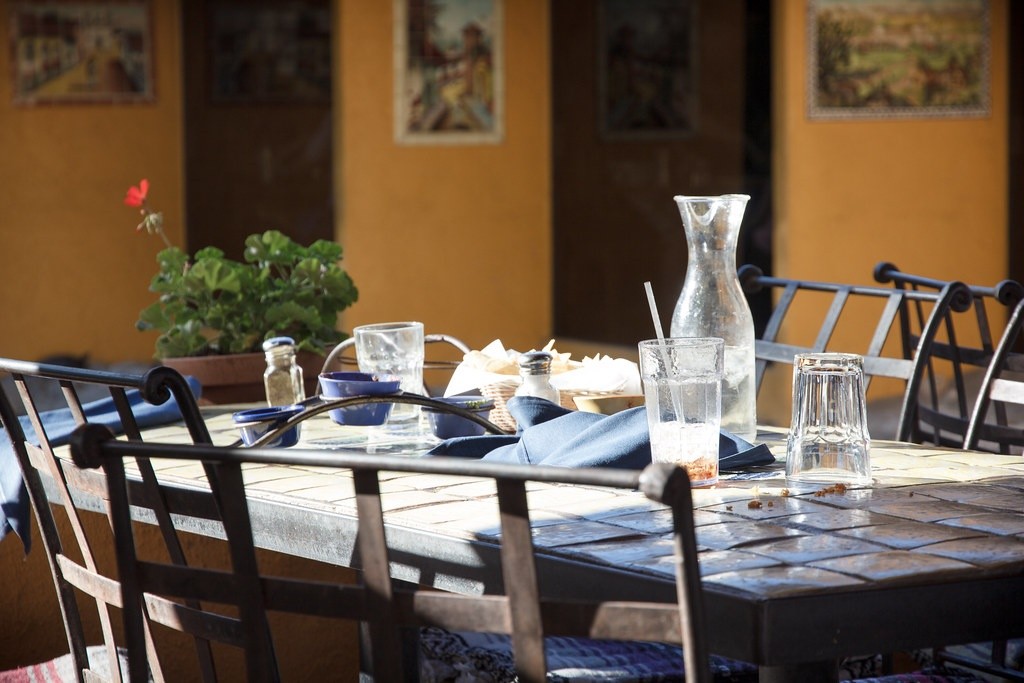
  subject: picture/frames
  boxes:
[804,0,992,120]
[392,0,506,146]
[8,0,160,108]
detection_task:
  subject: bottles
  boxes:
[510,353,561,413]
[258,335,306,408]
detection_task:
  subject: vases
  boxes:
[161,347,341,407]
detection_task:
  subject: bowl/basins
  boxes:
[318,370,404,428]
[571,394,646,416]
[419,396,495,440]
[231,404,305,449]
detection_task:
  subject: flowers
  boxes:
[123,179,360,358]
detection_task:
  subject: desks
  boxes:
[36,402,1024,683]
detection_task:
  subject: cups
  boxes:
[633,335,726,486]
[351,320,425,422]
[783,350,874,484]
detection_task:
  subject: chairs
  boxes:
[0,263,1024,683]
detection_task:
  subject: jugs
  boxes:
[668,189,759,444]
